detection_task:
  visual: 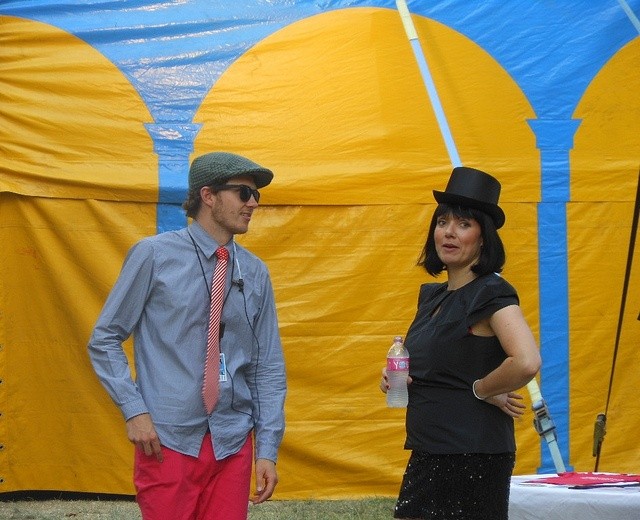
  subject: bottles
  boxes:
[386,336,410,408]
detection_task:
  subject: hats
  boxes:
[432,167,505,230]
[189,153,274,193]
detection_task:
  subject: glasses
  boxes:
[215,185,262,203]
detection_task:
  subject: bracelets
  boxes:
[473,380,486,401]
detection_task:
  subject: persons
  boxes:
[380,166,543,520]
[88,152,286,520]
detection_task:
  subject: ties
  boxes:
[201,246,229,416]
